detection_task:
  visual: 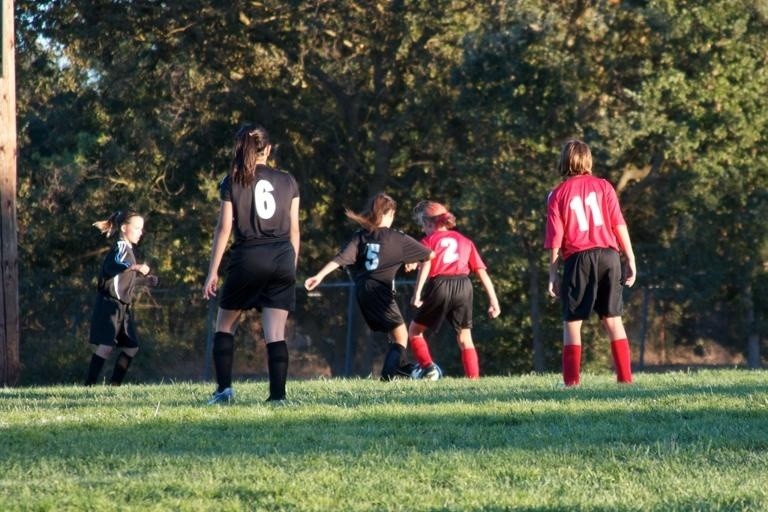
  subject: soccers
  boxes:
[411,361,443,380]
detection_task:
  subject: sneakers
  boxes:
[417,363,441,383]
[208,387,233,403]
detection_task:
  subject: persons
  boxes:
[81,208,158,385]
[542,139,639,386]
[198,123,302,403]
[408,200,502,379]
[301,193,437,383]
